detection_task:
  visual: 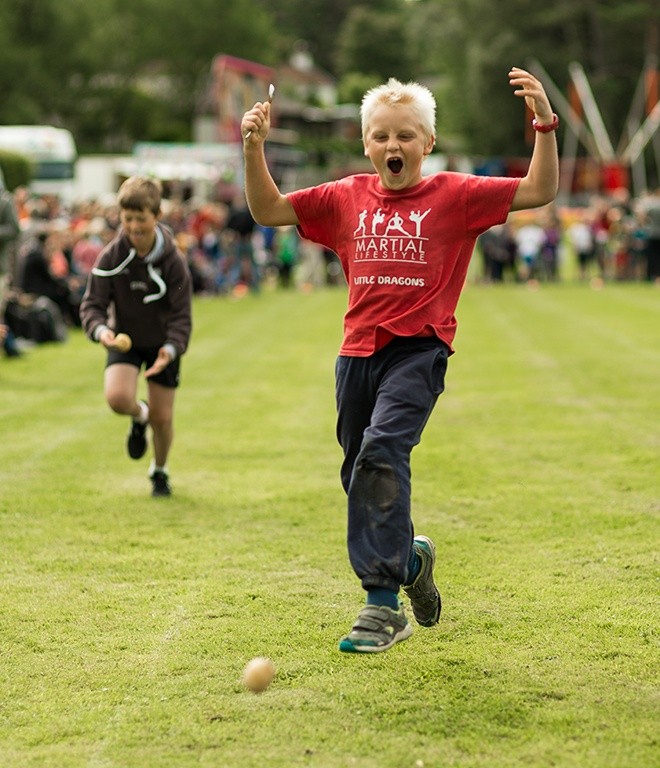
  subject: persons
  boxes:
[78,174,193,500]
[238,67,562,657]
[1,166,660,362]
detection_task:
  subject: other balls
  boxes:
[114,332,134,354]
[244,656,269,692]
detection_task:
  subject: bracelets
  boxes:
[531,114,560,133]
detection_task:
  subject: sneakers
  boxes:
[340,601,412,652]
[401,534,442,628]
[148,458,170,502]
[128,399,150,459]
